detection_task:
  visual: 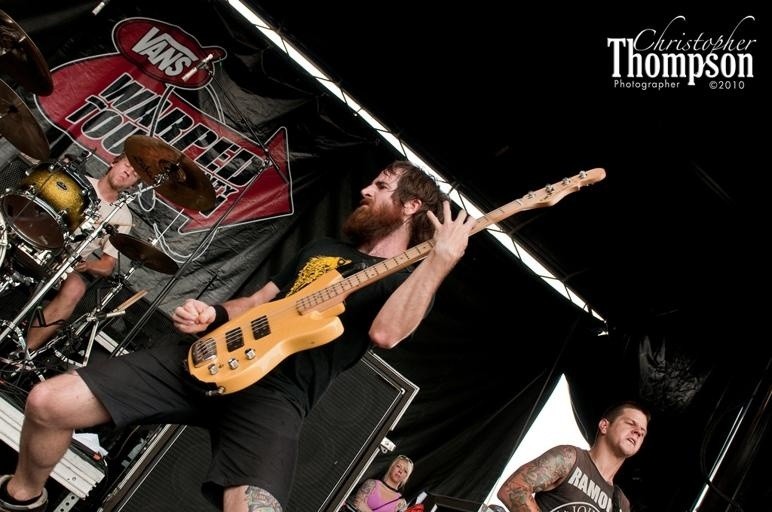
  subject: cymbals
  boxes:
[125,134,216,212]
[0,80,50,160]
[0,10,54,96]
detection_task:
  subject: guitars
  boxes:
[189,167,606,396]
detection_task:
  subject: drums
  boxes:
[1,159,100,251]
[1,211,16,268]
[7,229,74,275]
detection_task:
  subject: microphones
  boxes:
[180,52,213,82]
[86,310,125,322]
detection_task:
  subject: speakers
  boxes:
[83,348,422,512]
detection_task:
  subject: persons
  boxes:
[2,158,475,511]
[351,450,416,511]
[1,144,144,387]
[494,398,651,511]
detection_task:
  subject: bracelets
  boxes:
[208,301,231,328]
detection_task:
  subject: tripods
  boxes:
[0,262,141,383]
